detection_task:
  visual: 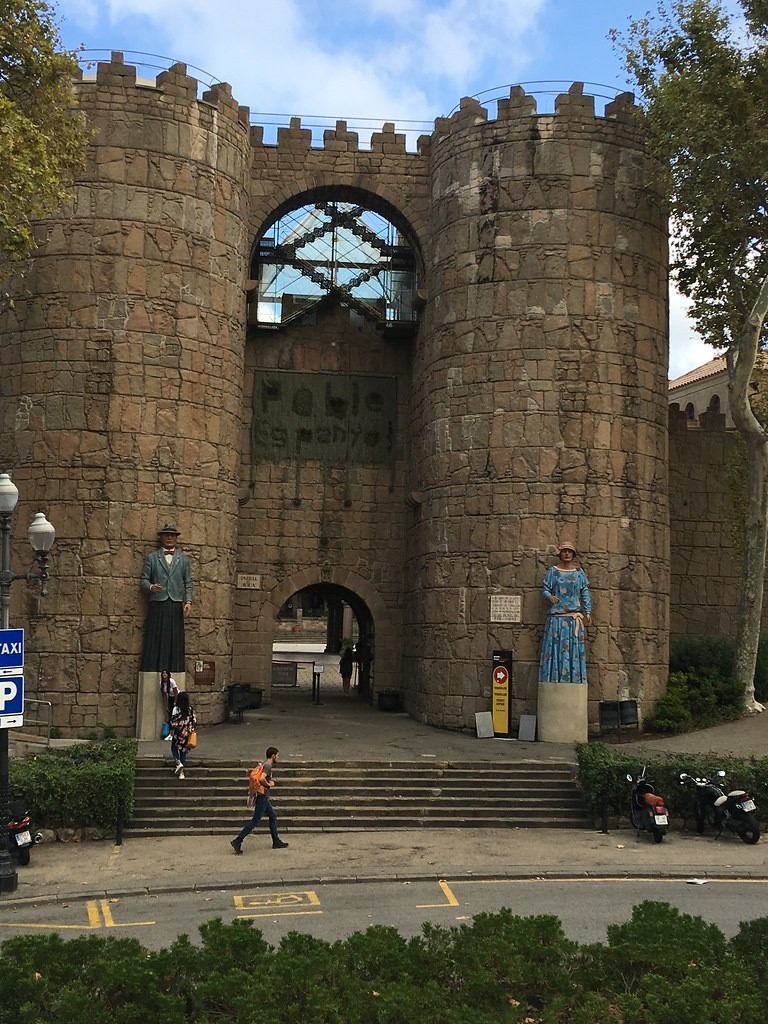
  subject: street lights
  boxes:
[0,472,56,893]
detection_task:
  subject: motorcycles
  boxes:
[679,769,762,845]
[6,799,44,866]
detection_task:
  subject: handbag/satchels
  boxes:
[188,732,196,748]
[160,722,170,738]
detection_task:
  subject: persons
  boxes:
[140,523,193,672]
[161,669,178,741]
[339,648,353,697]
[170,692,197,780]
[231,747,288,854]
[539,541,592,683]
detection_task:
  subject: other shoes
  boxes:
[175,763,184,774]
[165,734,173,741]
[272,838,289,848]
[179,774,185,779]
[231,839,243,854]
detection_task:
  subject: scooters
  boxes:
[626,765,670,843]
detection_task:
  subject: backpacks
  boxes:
[249,763,266,797]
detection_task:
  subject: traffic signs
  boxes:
[0,675,26,729]
[0,627,25,676]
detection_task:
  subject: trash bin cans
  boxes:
[376,689,400,711]
[228,683,265,712]
[598,698,639,725]
